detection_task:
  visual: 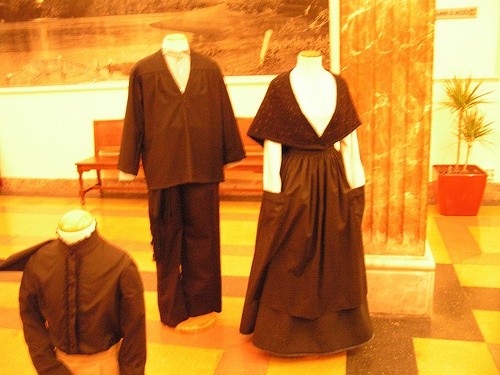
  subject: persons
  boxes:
[118,32,246,332]
[19,209,148,375]
[237,50,373,356]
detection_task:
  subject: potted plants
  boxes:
[432,76,495,217]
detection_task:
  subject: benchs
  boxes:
[74,117,264,206]
